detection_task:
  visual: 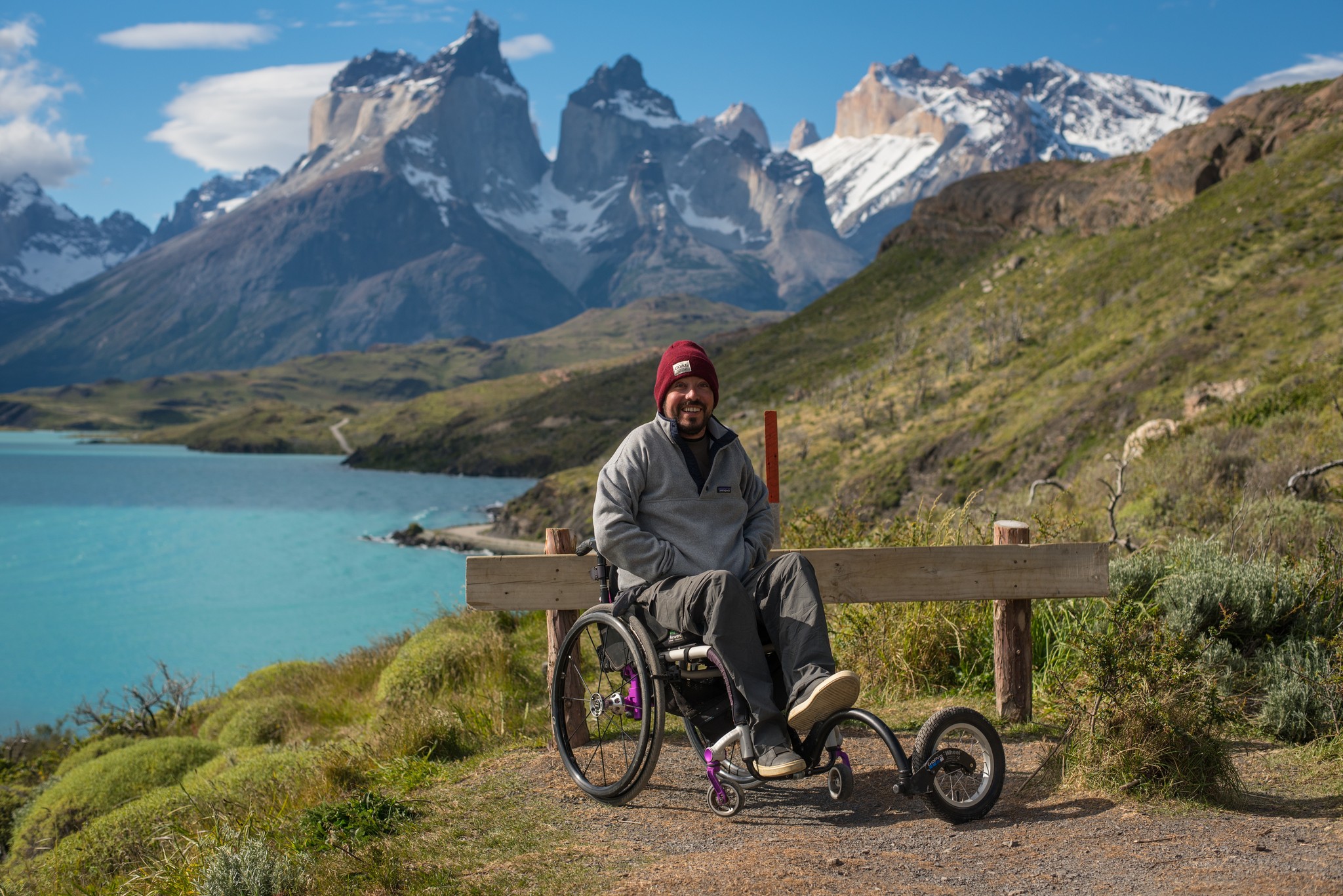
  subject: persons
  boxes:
[588,340,862,779]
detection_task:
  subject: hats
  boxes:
[654,340,719,413]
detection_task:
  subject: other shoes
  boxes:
[787,670,860,731]
[753,742,805,777]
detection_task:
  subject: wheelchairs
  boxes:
[548,537,1008,824]
